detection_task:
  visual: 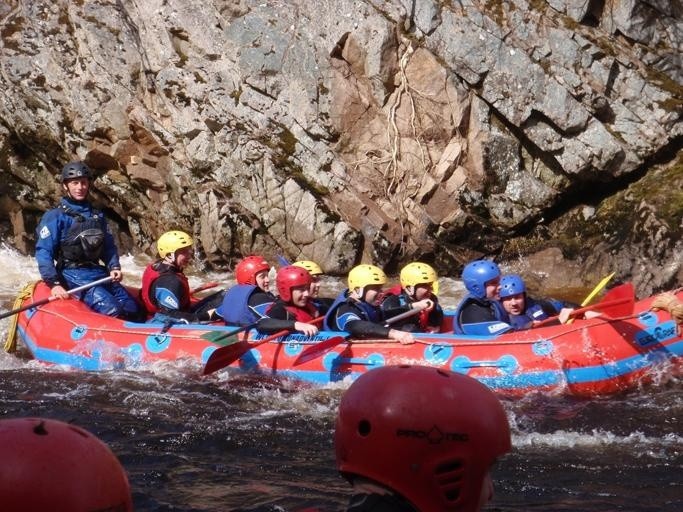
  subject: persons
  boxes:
[376,262,446,335]
[257,266,321,337]
[290,259,330,314]
[452,262,510,333]
[140,229,226,325]
[495,275,603,335]
[323,264,417,345]
[214,256,279,324]
[35,161,141,324]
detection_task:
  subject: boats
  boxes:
[5,281,683,398]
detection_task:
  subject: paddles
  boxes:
[564,270,621,321]
[291,306,428,365]
[530,283,636,329]
[204,316,326,375]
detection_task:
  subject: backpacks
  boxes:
[53,203,105,267]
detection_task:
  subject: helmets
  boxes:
[236,256,271,285]
[499,275,527,298]
[291,260,323,278]
[400,261,439,299]
[61,161,94,185]
[275,265,313,302]
[462,259,500,300]
[347,263,388,299]
[156,230,194,265]
